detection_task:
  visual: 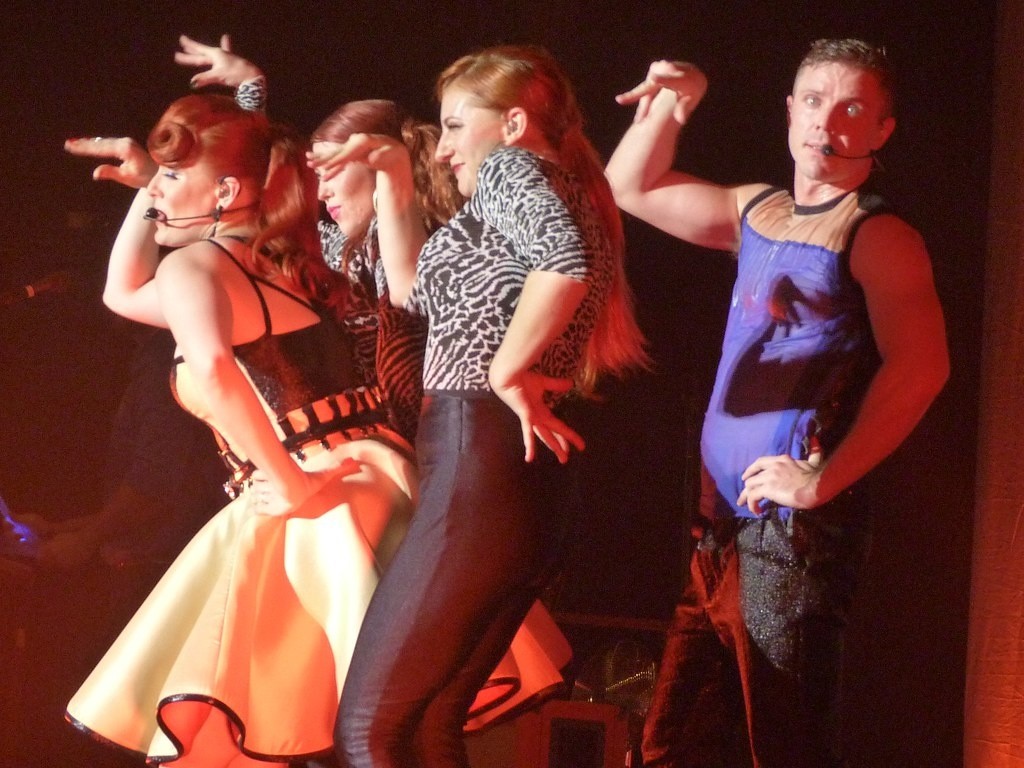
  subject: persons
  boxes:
[596,38,952,768]
[173,23,441,306]
[63,91,572,768]
[305,43,653,768]
[0,310,234,676]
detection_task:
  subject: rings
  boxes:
[93,136,103,143]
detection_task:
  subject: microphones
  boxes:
[821,141,888,160]
[142,207,221,223]
